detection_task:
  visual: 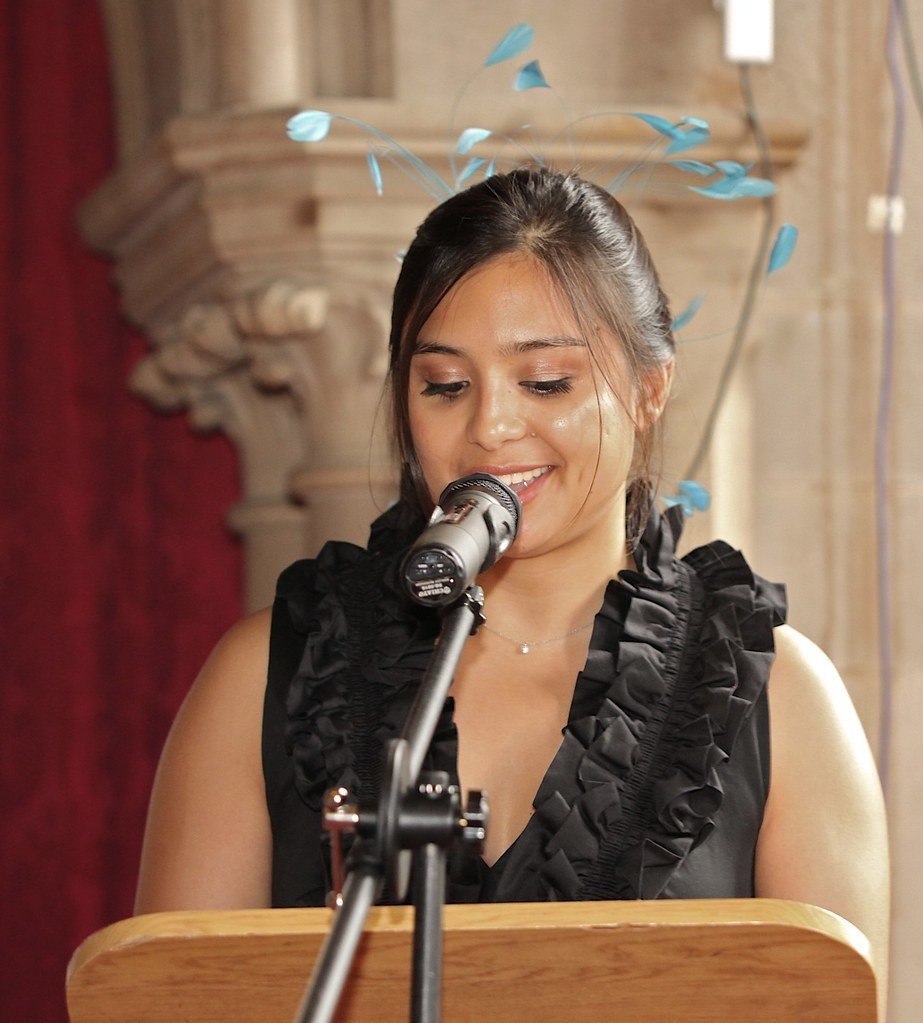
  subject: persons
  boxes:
[133,165,892,1023]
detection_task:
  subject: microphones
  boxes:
[395,473,521,608]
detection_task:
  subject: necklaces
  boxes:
[480,620,592,654]
